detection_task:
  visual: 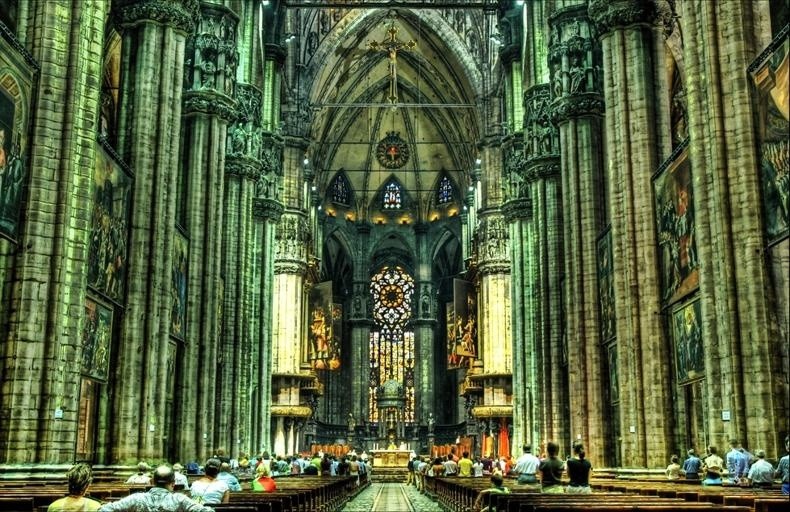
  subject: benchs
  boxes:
[404,457,789,512]
[1,457,372,510]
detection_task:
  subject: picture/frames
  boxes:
[169,221,190,344]
[81,296,116,383]
[746,22,790,250]
[607,340,621,407]
[595,226,616,345]
[166,340,179,404]
[651,137,699,312]
[1,19,41,246]
[672,296,705,387]
[86,131,135,308]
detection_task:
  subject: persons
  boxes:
[46,461,103,511]
[665,436,790,495]
[98,462,215,512]
[125,446,374,506]
[408,441,592,512]
